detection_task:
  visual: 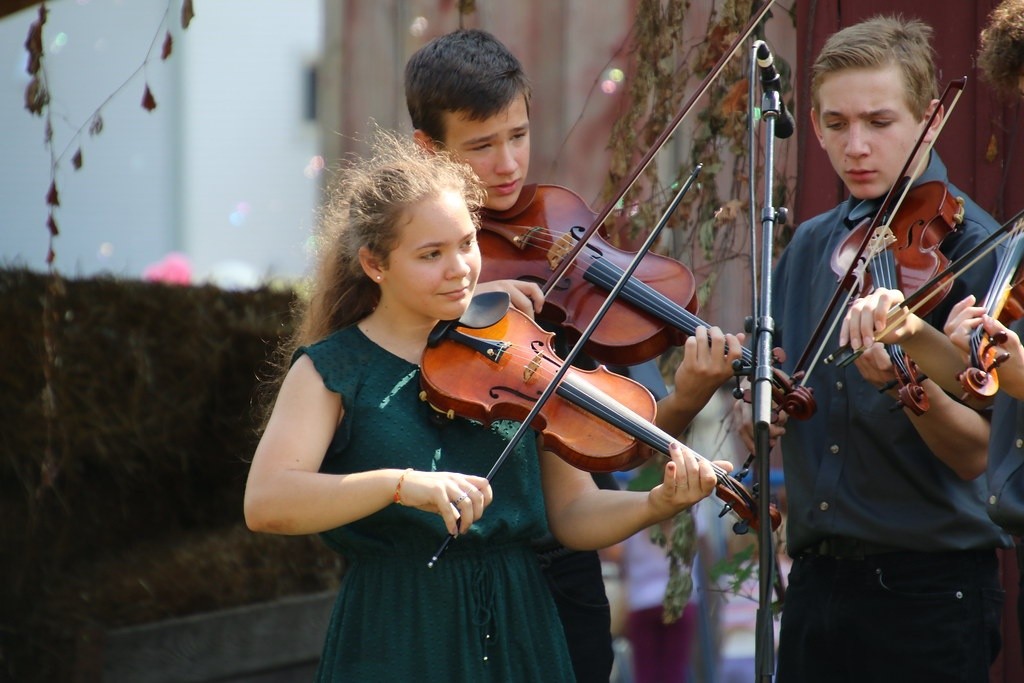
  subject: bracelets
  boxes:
[394,467,413,505]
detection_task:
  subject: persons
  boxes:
[404,29,747,683]
[242,125,736,683]
[745,1,1024,682]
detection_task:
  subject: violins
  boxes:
[419,292,782,541]
[473,182,819,422]
[825,182,967,416]
[955,219,1024,405]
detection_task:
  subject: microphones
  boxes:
[755,39,796,139]
